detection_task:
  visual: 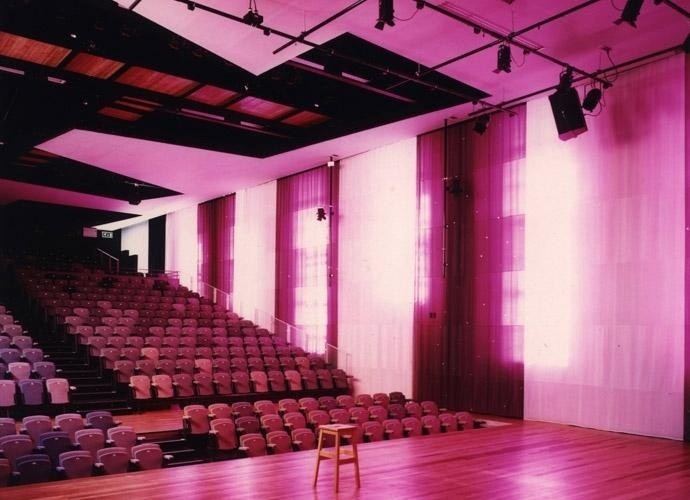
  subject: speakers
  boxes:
[549,88,588,140]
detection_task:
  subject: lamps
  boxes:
[582,88,601,113]
[472,114,490,134]
[445,175,467,195]
[374,2,395,30]
[612,1,645,27]
[493,45,513,73]
[316,208,327,221]
[327,157,335,168]
